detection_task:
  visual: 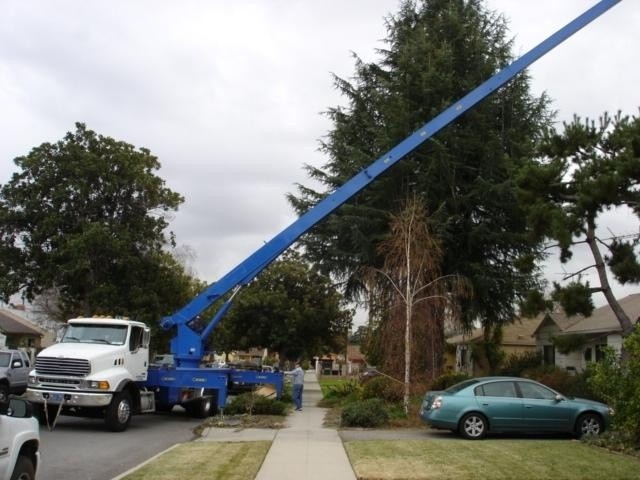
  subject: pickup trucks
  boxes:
[0,349,35,403]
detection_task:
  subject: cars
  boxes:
[0,387,40,480]
[419,374,615,444]
[203,360,274,373]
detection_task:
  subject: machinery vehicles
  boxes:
[22,0,621,442]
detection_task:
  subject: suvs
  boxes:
[149,353,173,370]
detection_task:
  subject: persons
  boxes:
[284,362,305,411]
